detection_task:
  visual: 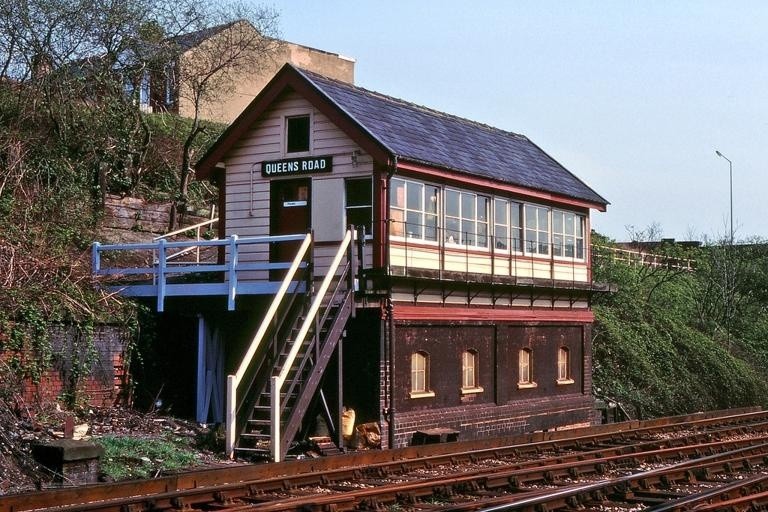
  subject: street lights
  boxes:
[714,150,734,295]
